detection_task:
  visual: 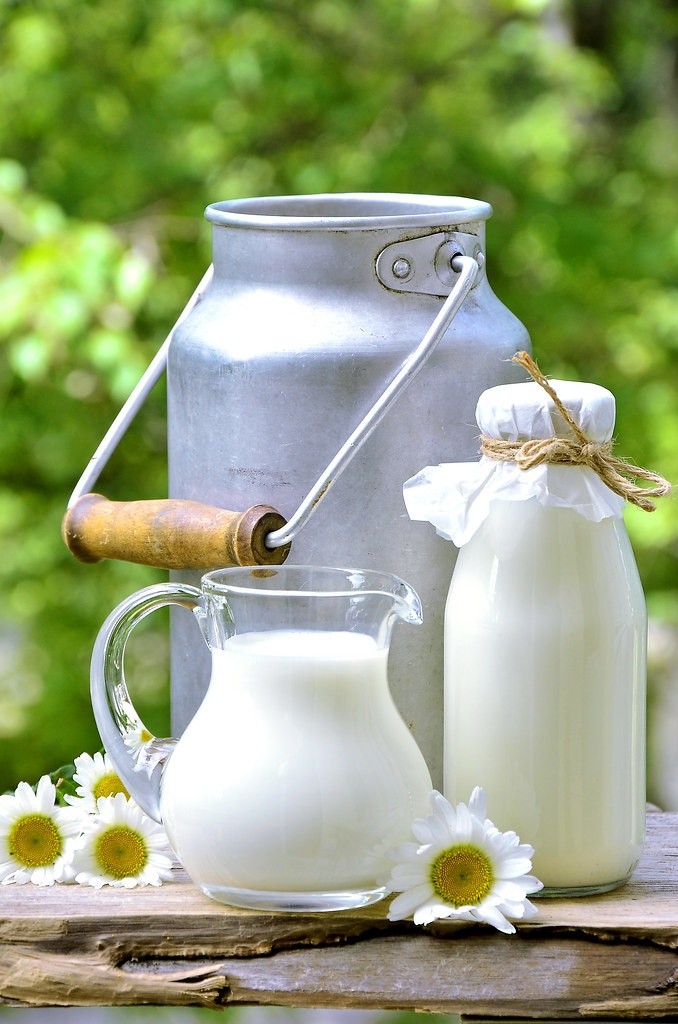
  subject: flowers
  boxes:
[122,721,168,781]
[0,752,175,887]
[385,787,544,934]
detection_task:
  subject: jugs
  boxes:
[91,563,435,914]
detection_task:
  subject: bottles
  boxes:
[61,193,534,795]
[443,379,647,895]
